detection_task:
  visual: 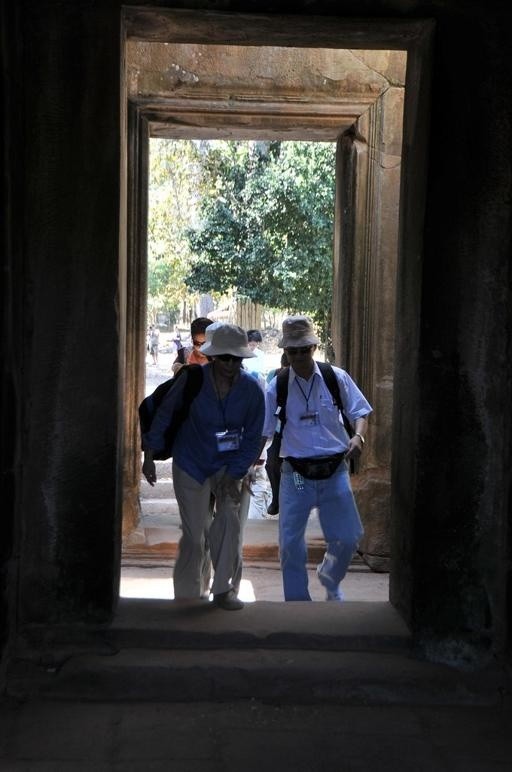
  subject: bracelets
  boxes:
[356,433,365,447]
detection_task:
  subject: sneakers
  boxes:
[214,589,245,610]
[326,585,344,601]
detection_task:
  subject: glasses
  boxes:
[287,346,313,356]
[192,339,205,346]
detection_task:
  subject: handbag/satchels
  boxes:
[283,453,346,479]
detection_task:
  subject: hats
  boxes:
[278,315,320,349]
[199,321,258,358]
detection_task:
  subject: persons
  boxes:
[265,353,290,514]
[146,316,269,598]
[140,320,264,610]
[262,314,374,601]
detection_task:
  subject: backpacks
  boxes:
[138,364,203,460]
